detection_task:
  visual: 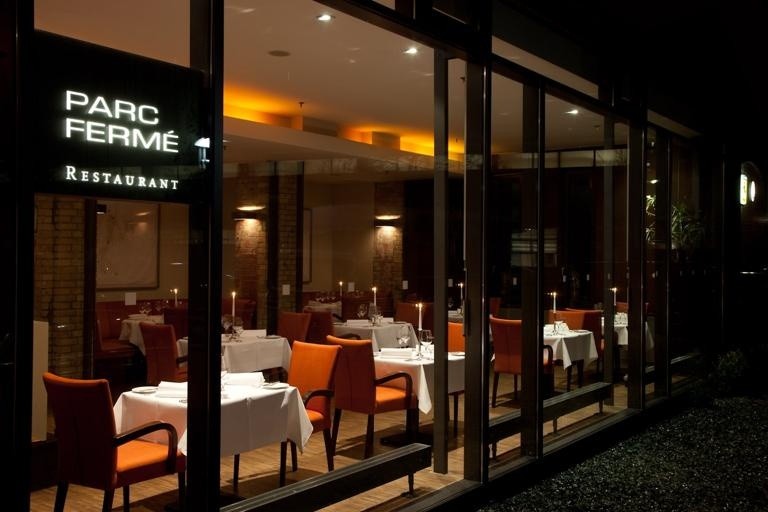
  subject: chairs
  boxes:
[163,306,188,339]
[488,295,502,320]
[42,370,186,511]
[95,317,135,367]
[138,322,186,385]
[239,287,437,344]
[448,303,651,407]
[275,340,343,472]
[326,332,413,463]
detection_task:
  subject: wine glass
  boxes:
[355,303,381,324]
[419,329,431,360]
[447,298,453,313]
[315,290,336,304]
[221,313,244,343]
[395,324,412,355]
[129,299,167,320]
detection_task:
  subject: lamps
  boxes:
[232,210,261,222]
[374,218,399,229]
[738,170,758,210]
[96,203,108,216]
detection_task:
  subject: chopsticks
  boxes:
[260,383,289,389]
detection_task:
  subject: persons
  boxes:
[567,277,593,308]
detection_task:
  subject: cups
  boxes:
[544,321,569,336]
[612,312,628,325]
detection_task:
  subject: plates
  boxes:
[575,329,587,332]
[132,386,159,394]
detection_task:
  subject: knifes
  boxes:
[263,380,280,386]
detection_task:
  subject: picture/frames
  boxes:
[96,198,161,292]
[301,207,314,285]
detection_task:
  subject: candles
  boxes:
[612,287,618,306]
[172,287,181,306]
[229,290,239,320]
[459,282,465,298]
[339,281,343,295]
[552,291,558,314]
